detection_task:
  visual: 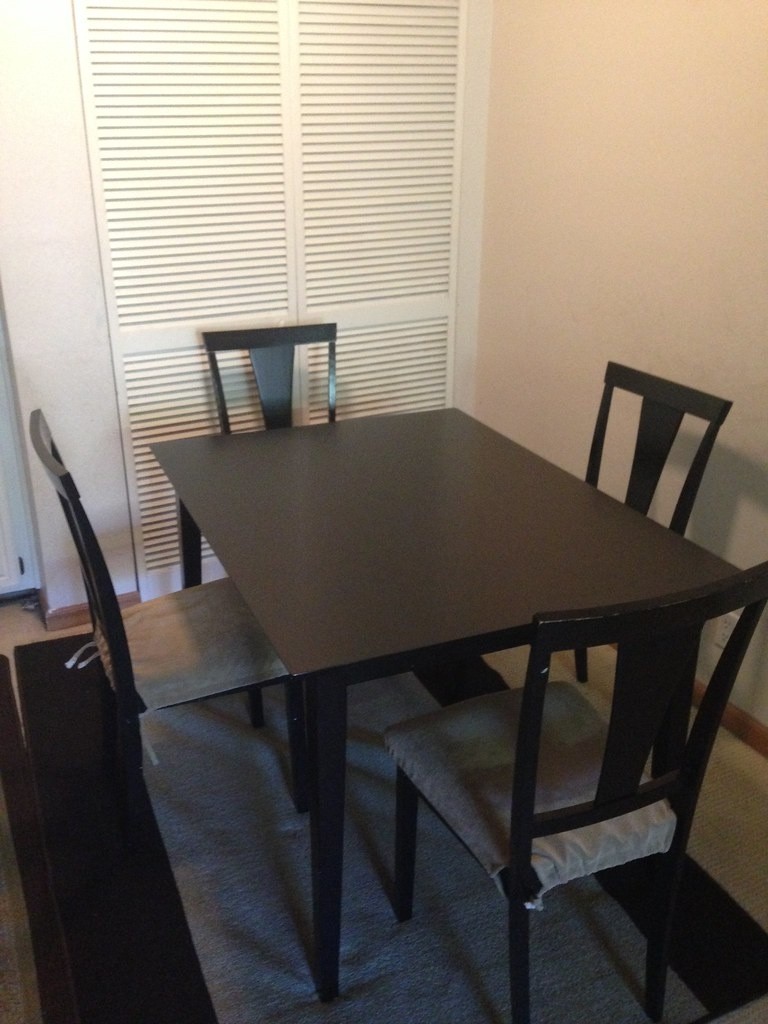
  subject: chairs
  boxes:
[201,323,337,432]
[380,562,767,1024]
[29,410,310,873]
[569,361,735,682]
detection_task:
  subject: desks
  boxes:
[150,406,744,1002]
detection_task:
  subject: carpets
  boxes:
[0,627,768,1024]
